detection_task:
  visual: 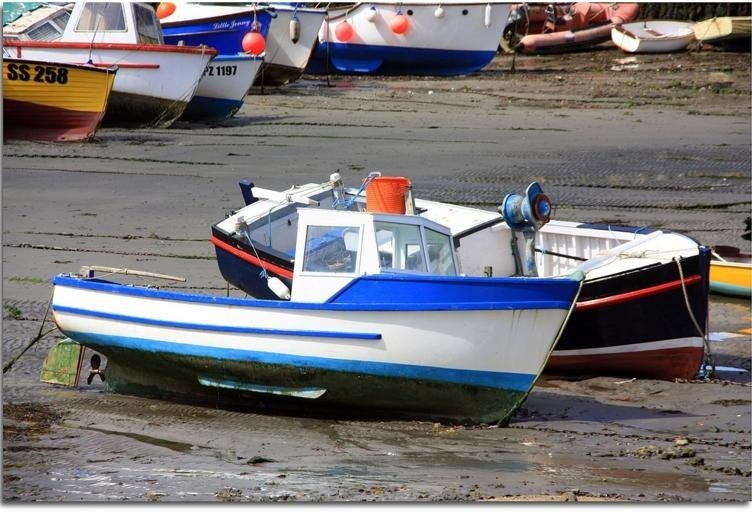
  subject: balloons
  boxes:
[241,31,266,56]
[335,23,353,42]
[434,7,444,20]
[365,10,377,23]
[390,15,409,34]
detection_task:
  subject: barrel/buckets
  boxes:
[360,176,411,215]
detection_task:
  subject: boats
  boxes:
[47,208,582,433]
[697,219,752,299]
[206,169,713,383]
